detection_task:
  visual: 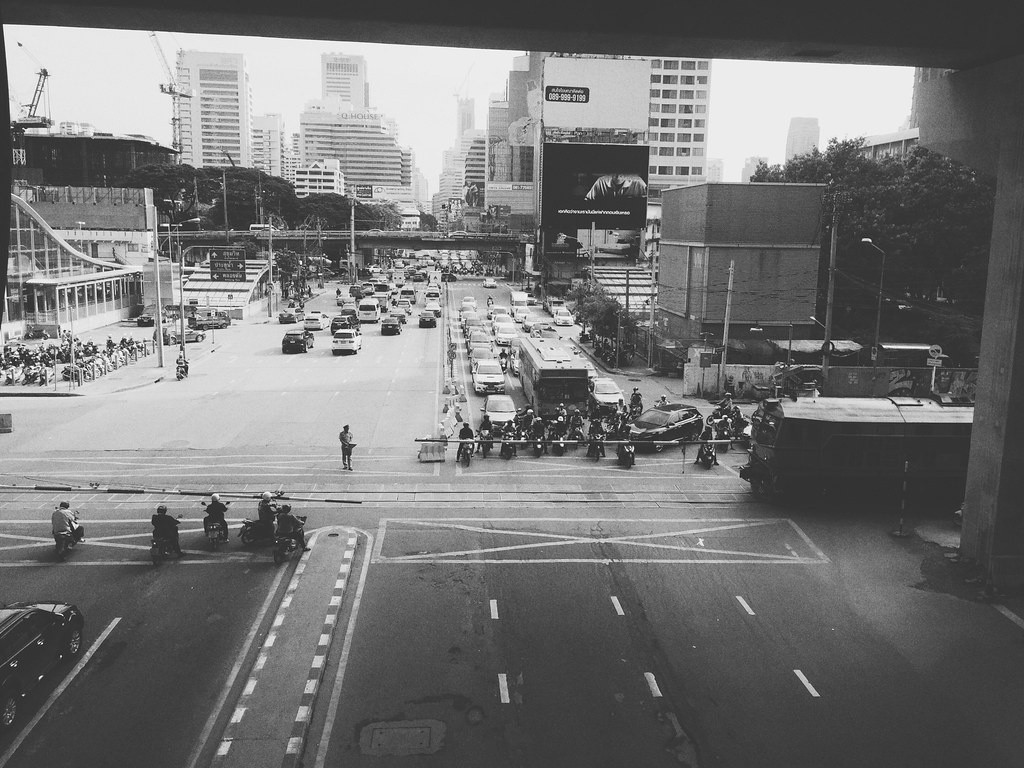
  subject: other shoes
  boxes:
[224,539,230,543]
[304,547,311,550]
[714,462,719,465]
[694,461,698,464]
[343,466,348,469]
[349,466,353,470]
[454,445,570,463]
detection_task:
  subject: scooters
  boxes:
[696,404,748,467]
[149,514,184,567]
[461,421,637,469]
[177,358,191,381]
[198,499,311,564]
[0,325,144,386]
[581,332,635,367]
[53,506,79,561]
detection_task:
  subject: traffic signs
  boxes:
[210,249,245,283]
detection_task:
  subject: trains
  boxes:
[740,392,978,503]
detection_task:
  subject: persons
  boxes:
[0,330,136,364]
[50,501,86,543]
[335,288,341,297]
[499,347,508,359]
[202,493,230,544]
[453,386,745,465]
[175,353,189,375]
[391,298,397,306]
[338,423,354,471]
[151,505,187,559]
[257,491,312,552]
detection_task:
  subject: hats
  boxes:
[343,424,350,428]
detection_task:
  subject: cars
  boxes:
[282,249,574,377]
[625,405,703,455]
[479,394,518,434]
[138,304,169,327]
[153,324,207,345]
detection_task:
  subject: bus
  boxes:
[519,338,625,420]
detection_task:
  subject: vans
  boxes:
[191,309,232,330]
[249,224,280,232]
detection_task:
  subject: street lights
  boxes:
[612,232,656,370]
[808,315,832,396]
[75,222,86,275]
[154,217,201,368]
[559,221,594,285]
[861,237,887,367]
[750,323,794,373]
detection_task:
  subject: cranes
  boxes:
[147,30,195,163]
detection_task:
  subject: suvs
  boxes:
[587,378,624,419]
[0,599,85,734]
[473,359,509,397]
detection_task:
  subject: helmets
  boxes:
[211,493,220,502]
[282,503,291,513]
[702,390,740,434]
[614,385,667,432]
[461,400,599,438]
[179,353,183,358]
[59,500,70,508]
[157,505,168,514]
[262,491,272,501]
